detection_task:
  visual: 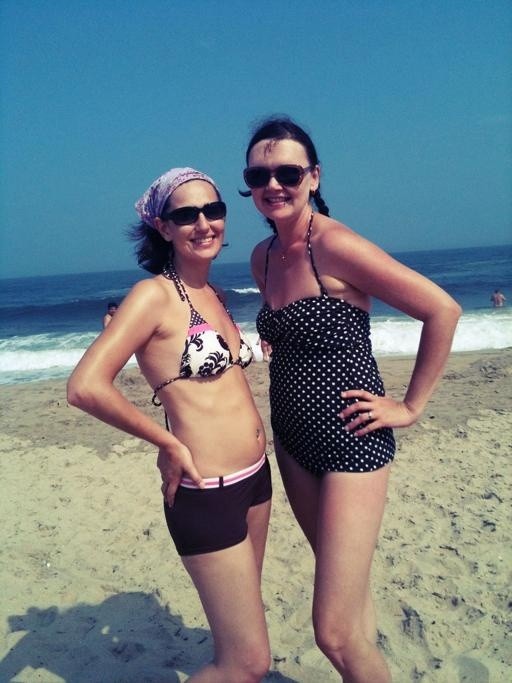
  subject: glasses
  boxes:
[163,201,226,225]
[243,165,312,186]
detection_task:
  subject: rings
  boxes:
[367,411,373,421]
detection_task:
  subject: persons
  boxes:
[242,116,464,682]
[490,290,507,308]
[68,162,274,680]
[103,301,118,330]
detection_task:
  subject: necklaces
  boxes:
[276,222,309,265]
